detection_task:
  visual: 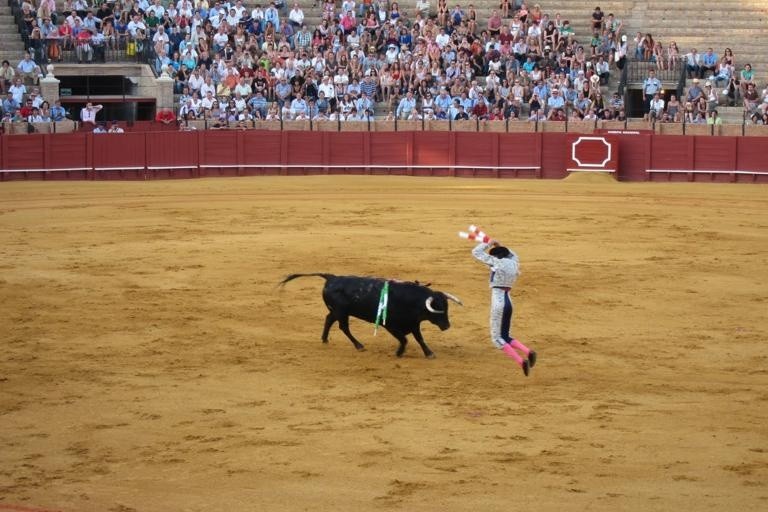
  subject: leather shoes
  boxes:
[528,351,537,368]
[521,359,530,377]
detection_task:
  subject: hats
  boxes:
[487,246,510,257]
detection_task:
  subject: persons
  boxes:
[2,1,767,133]
[470,237,537,377]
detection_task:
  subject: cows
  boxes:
[275,271,464,361]
